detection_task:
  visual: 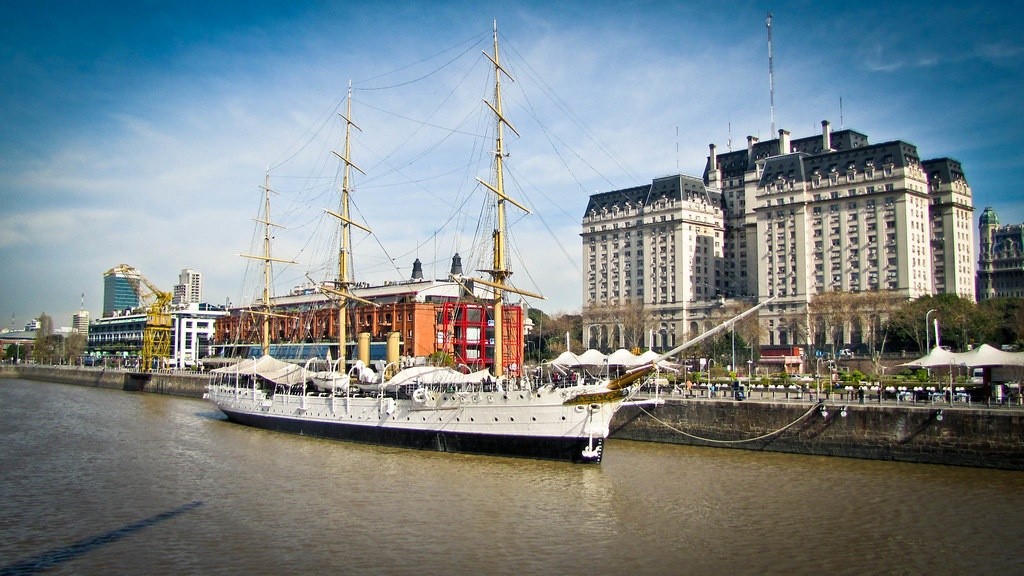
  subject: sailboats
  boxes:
[201,20,656,465]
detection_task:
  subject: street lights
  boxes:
[732,318,744,372]
[588,324,601,350]
[926,309,939,355]
[683,359,693,399]
[708,359,717,400]
[817,358,823,403]
[827,360,834,394]
[527,341,535,359]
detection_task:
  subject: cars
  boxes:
[1001,344,1013,352]
[940,346,951,352]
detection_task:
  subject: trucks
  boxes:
[816,349,854,359]
